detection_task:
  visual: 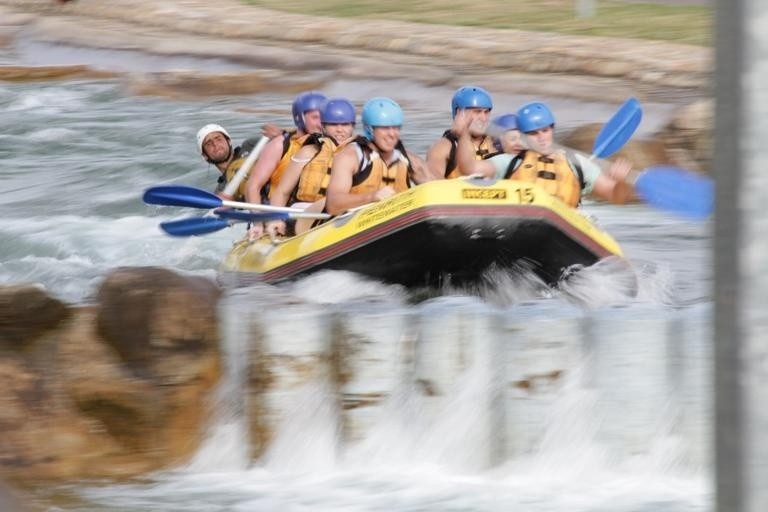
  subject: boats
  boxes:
[215,172,639,309]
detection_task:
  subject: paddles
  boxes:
[589,98,641,158]
[469,123,713,217]
[142,185,334,236]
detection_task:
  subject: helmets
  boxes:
[362,96,405,142]
[452,86,493,121]
[196,124,231,154]
[491,103,555,151]
[292,94,355,133]
